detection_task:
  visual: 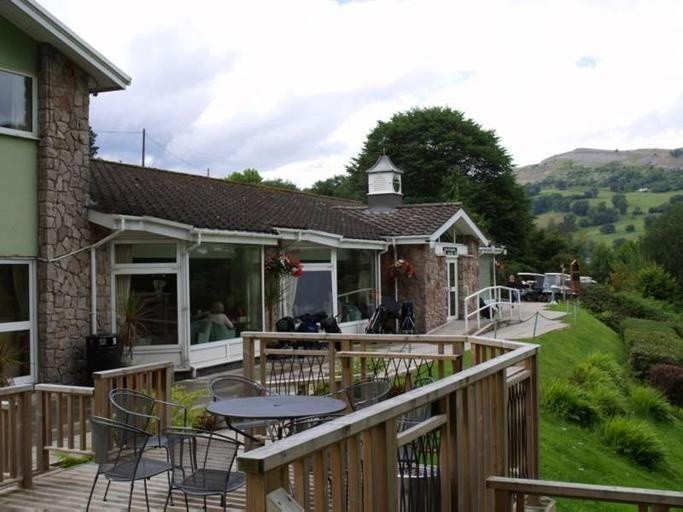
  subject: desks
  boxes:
[206,394,348,441]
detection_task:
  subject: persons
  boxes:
[504,273,529,302]
[146,287,366,345]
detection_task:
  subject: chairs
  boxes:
[396,376,439,474]
[86,413,190,512]
[478,298,495,319]
[324,377,392,413]
[110,388,188,506]
[266,300,345,353]
[365,299,418,334]
[203,375,279,472]
[277,414,355,503]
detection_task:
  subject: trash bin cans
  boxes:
[85,333,121,387]
[401,302,415,334]
